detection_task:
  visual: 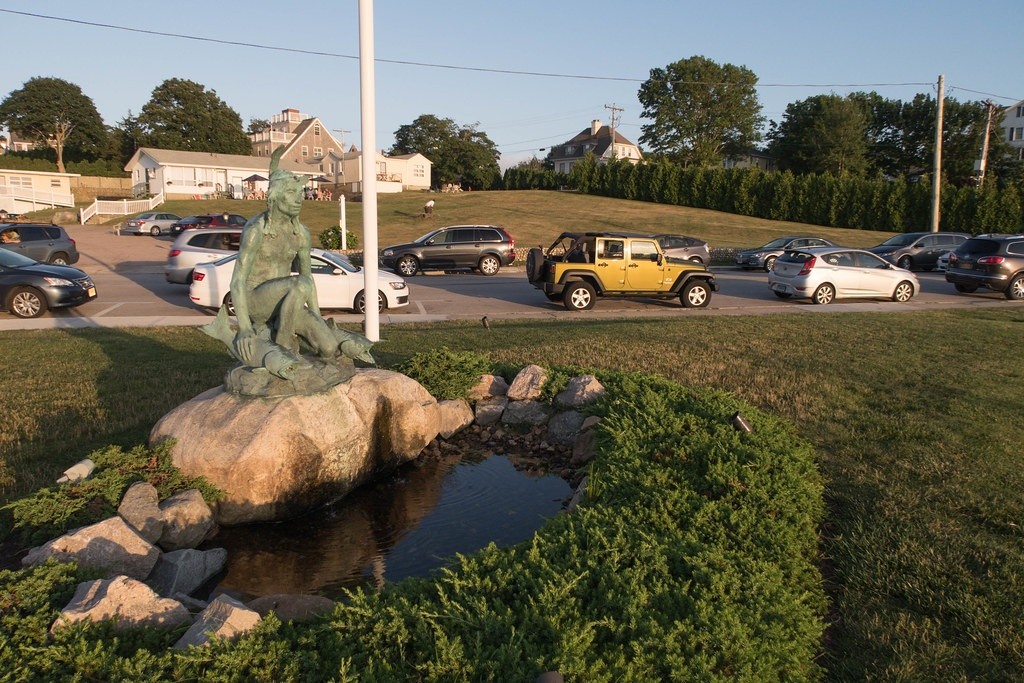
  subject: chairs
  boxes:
[797,240,804,246]
[609,244,621,251]
[324,193,332,201]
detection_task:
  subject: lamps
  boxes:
[222,210,230,222]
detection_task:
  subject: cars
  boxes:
[188,247,410,315]
[768,247,921,307]
[0,248,98,319]
[646,233,712,268]
[734,233,852,274]
[860,229,973,271]
[170,212,250,240]
[936,232,988,272]
[125,210,183,236]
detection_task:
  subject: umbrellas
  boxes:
[242,175,270,192]
[309,175,332,192]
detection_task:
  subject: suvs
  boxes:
[0,221,80,266]
[378,224,516,277]
[526,230,721,311]
[944,233,1024,301]
[163,226,243,284]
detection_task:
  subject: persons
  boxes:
[229,169,340,370]
[441,182,472,194]
[423,199,435,219]
[243,186,333,201]
[1,231,20,244]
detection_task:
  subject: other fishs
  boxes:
[196,304,298,380]
[323,317,375,364]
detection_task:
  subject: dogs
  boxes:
[113,222,122,236]
